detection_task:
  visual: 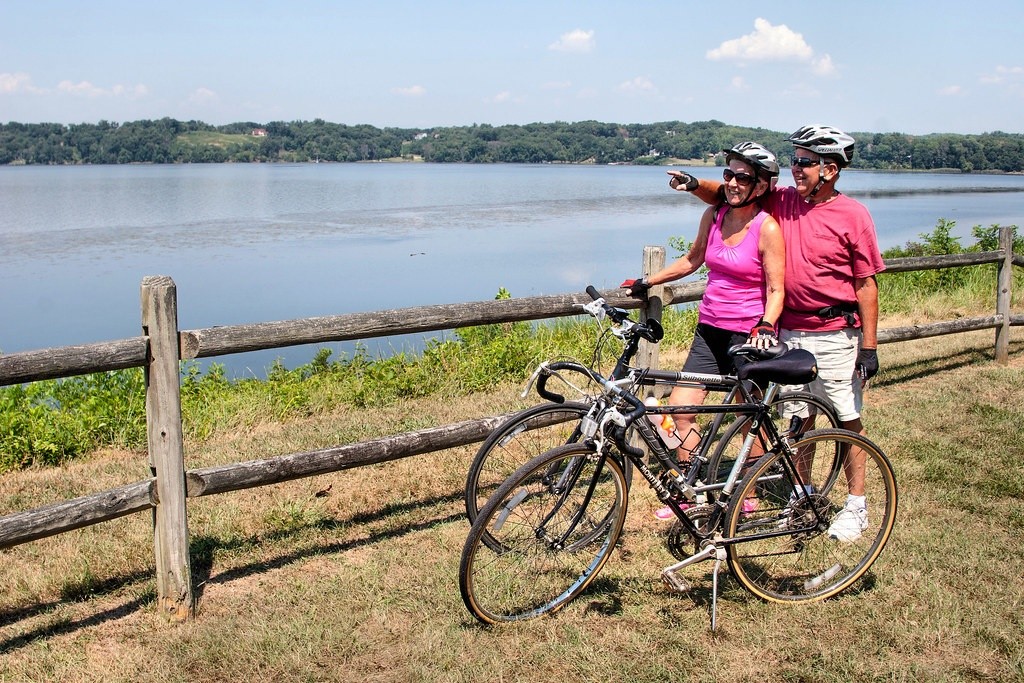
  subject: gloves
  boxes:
[746,320,778,351]
[620,276,652,298]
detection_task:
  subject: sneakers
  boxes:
[653,495,705,522]
[776,495,811,531]
[824,506,868,543]
[739,494,758,522]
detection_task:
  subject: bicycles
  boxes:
[464,283,844,530]
[460,347,900,637]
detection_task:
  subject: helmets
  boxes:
[787,124,856,169]
[723,140,779,183]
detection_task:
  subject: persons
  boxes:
[666,124,887,543]
[622,141,786,520]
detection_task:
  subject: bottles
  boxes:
[644,396,681,449]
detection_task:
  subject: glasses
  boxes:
[789,154,832,169]
[722,168,761,187]
